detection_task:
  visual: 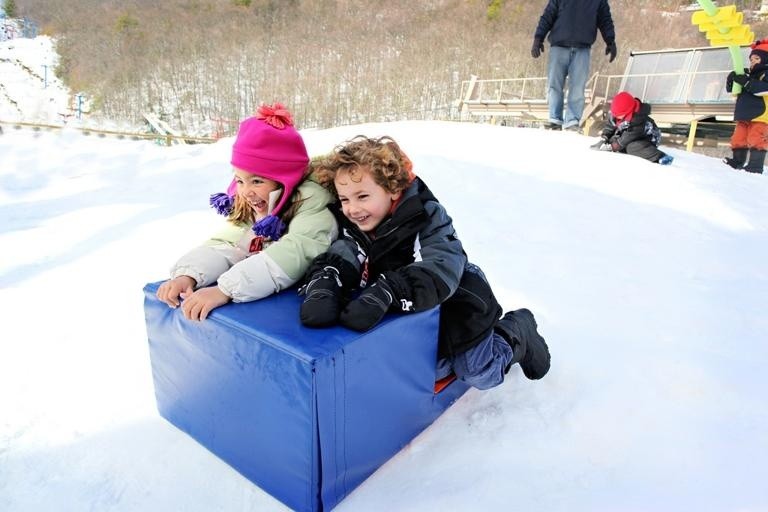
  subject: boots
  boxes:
[494,307,551,381]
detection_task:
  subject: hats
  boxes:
[610,91,636,116]
[749,40,768,63]
[209,103,312,238]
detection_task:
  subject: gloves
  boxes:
[726,70,735,92]
[604,41,617,63]
[734,74,752,90]
[591,137,613,152]
[341,272,396,332]
[298,252,353,331]
[530,40,544,59]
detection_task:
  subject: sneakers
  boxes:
[722,156,744,171]
[740,165,764,175]
[543,120,583,135]
[659,155,675,166]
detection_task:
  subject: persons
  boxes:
[722,37,768,175]
[592,91,673,165]
[155,102,342,321]
[531,0,617,134]
[298,134,551,391]
